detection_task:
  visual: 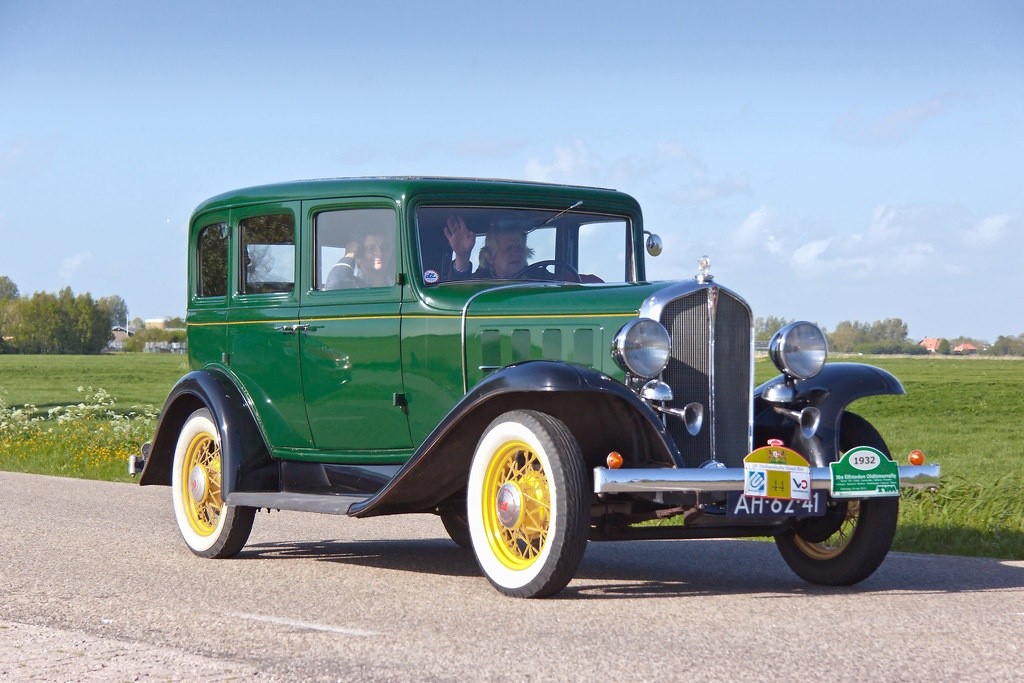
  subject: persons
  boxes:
[439,210,548,282]
[325,225,393,291]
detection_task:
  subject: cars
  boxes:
[122,174,944,599]
